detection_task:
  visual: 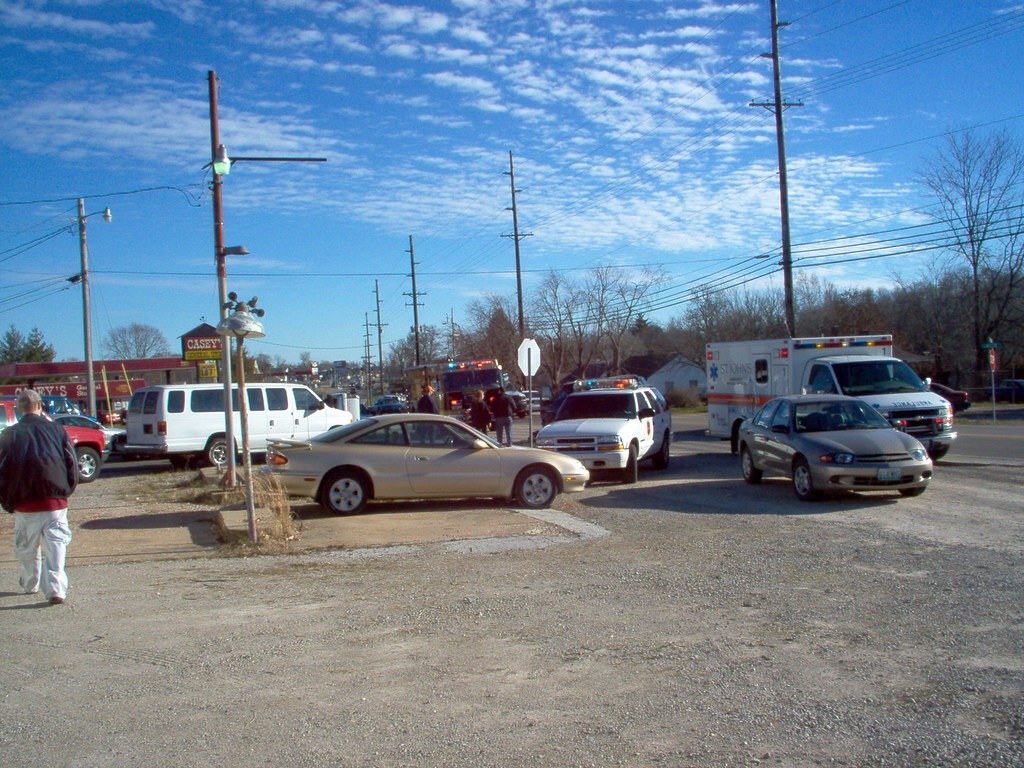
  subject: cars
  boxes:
[923,381,970,417]
[0,394,129,484]
[521,388,548,413]
[737,393,934,502]
[265,413,590,517]
[371,397,402,416]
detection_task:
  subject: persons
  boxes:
[470,390,492,435]
[0,390,78,604]
[491,388,515,447]
[417,385,439,445]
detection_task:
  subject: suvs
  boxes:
[533,375,674,484]
[504,382,528,418]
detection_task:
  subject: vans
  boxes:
[123,379,355,470]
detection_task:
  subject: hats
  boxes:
[18,390,45,407]
[425,386,434,391]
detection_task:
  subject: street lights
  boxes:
[216,290,269,543]
[207,142,250,489]
[75,198,113,419]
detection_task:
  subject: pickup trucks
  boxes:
[968,377,1024,404]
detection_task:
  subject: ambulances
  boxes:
[700,334,959,459]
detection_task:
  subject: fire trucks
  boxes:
[403,357,511,428]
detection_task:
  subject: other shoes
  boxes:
[19,576,38,594]
[49,596,65,604]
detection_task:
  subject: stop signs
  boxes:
[988,349,997,373]
